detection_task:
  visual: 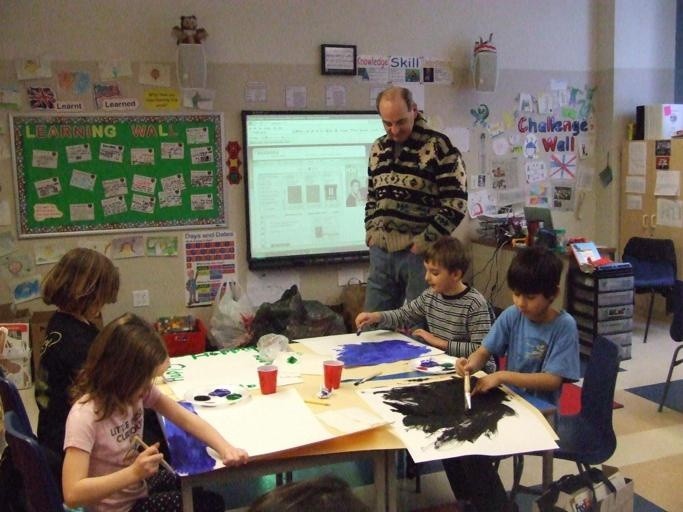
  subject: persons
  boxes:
[346,179,362,209]
[358,86,467,337]
[58,311,249,512]
[442,244,580,512]
[32,247,120,452]
[354,237,495,374]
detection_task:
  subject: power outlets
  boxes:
[131,289,149,307]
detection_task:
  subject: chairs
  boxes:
[519,331,623,498]
[621,237,681,344]
[0,375,35,439]
[0,406,61,512]
[656,278,683,414]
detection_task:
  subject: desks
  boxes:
[152,328,557,512]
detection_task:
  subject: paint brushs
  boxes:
[353,370,383,386]
[357,327,362,336]
[134,435,177,476]
[464,371,472,416]
[304,400,330,407]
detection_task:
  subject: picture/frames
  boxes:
[320,44,358,76]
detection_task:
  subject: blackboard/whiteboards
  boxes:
[9,113,229,239]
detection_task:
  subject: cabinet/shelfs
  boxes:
[620,139,683,317]
[472,238,573,311]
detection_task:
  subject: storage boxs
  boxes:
[33,310,103,379]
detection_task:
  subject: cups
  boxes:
[256,365,278,395]
[322,360,344,389]
